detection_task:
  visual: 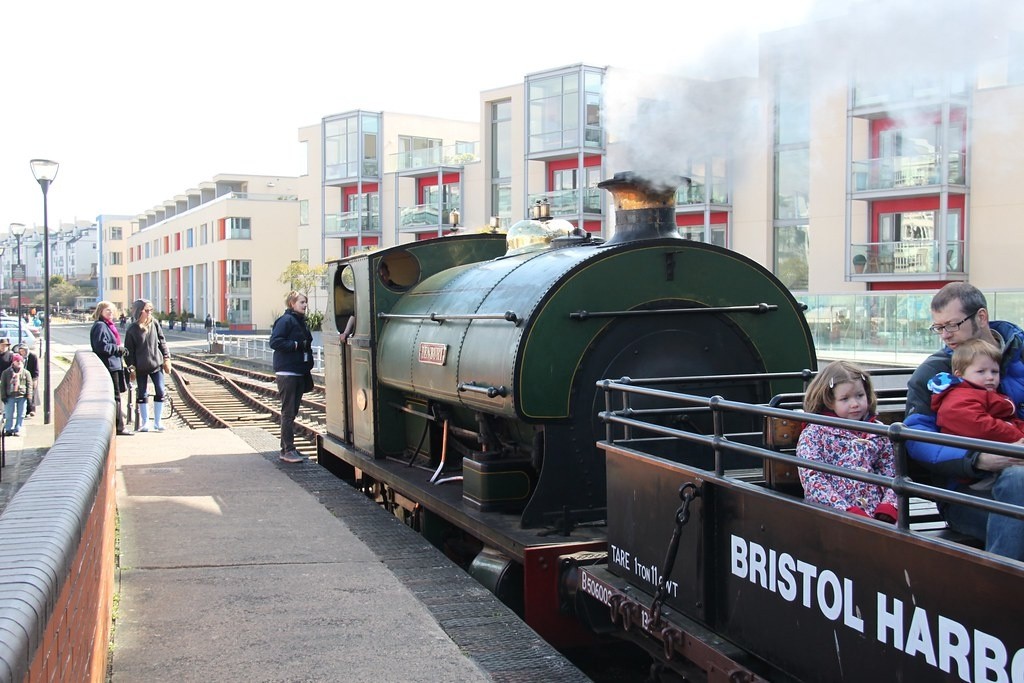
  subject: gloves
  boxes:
[1,398,8,404]
[162,358,171,374]
[129,366,136,381]
[118,346,130,357]
[295,339,310,352]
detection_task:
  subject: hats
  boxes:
[11,353,23,362]
[0,339,11,345]
[18,343,29,357]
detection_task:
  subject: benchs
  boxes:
[763,388,978,545]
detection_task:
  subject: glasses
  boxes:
[930,312,977,334]
[142,309,154,314]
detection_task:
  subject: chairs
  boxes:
[947,250,955,271]
[909,248,928,271]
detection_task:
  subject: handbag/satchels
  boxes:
[303,371,314,393]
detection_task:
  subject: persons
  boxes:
[340,261,399,342]
[119,314,126,328]
[902,282,1024,563]
[931,337,1024,443]
[23,313,41,327]
[124,300,171,431]
[269,290,314,462]
[0,338,40,436]
[204,313,213,342]
[796,361,898,525]
[90,301,134,435]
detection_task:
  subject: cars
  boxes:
[0,309,53,355]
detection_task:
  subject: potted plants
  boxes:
[853,254,867,274]
[278,196,287,200]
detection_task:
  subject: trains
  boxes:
[315,195,1024,683]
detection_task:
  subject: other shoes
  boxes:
[117,430,133,436]
[30,412,35,417]
[280,451,309,460]
[14,431,20,437]
[284,451,304,463]
[25,414,29,418]
[3,431,11,436]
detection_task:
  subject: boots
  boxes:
[137,403,149,433]
[153,401,165,431]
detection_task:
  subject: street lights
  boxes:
[29,158,60,424]
[9,222,28,343]
